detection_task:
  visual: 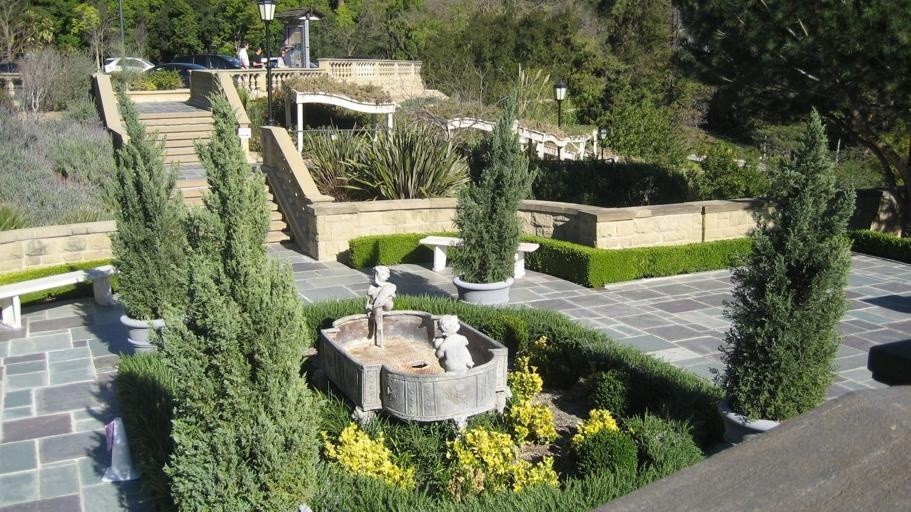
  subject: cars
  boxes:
[140,62,206,87]
[257,58,317,68]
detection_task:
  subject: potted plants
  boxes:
[707,104,860,446]
[99,84,192,353]
[451,89,539,309]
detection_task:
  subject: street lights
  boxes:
[256,1,275,126]
[599,129,607,161]
[552,81,567,166]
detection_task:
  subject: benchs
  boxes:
[0,264,120,329]
[418,235,540,278]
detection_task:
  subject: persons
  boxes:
[283,46,295,68]
[366,266,397,311]
[238,39,250,69]
[435,315,475,372]
[277,50,289,68]
[253,46,263,68]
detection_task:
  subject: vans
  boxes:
[171,54,246,69]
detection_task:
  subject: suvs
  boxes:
[101,57,153,74]
